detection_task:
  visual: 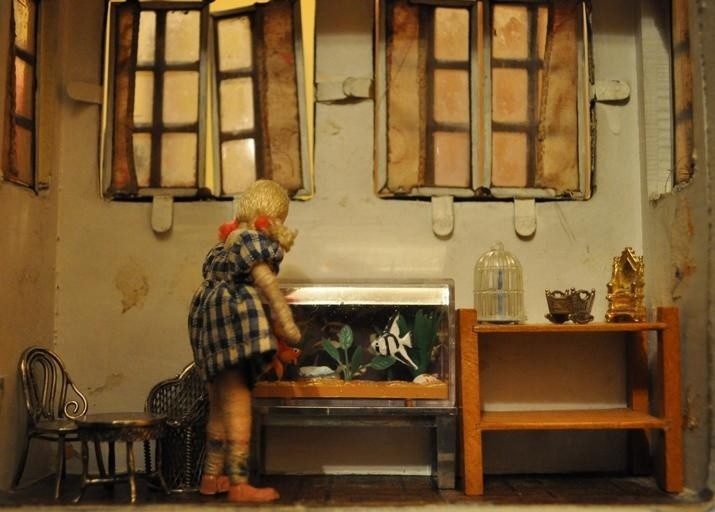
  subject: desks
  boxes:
[70,413,168,505]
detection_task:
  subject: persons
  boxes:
[186,178,305,503]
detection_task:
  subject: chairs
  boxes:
[145,361,219,494]
[12,346,88,501]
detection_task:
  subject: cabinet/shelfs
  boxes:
[455,305,685,496]
[250,399,455,488]
